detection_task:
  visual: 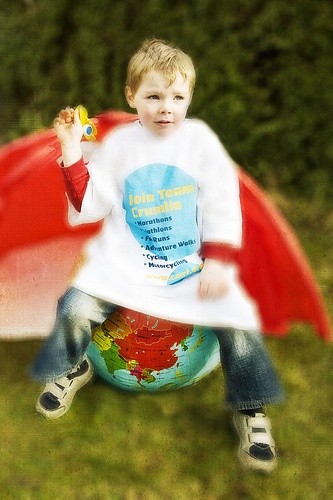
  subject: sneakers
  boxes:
[36,354,92,419]
[233,410,277,478]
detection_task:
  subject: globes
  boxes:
[83,306,222,392]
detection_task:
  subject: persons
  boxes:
[31,41,282,472]
